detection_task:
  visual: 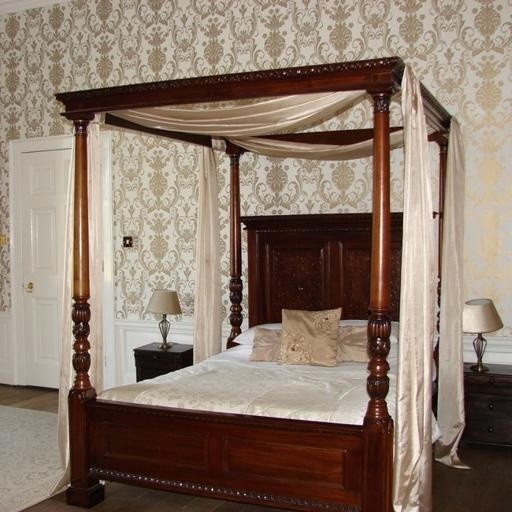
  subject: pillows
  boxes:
[232,307,399,367]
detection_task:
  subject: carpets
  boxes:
[1,404,71,512]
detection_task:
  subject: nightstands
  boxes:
[457,361,511,449]
[134,342,193,381]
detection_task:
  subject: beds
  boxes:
[52,56,460,510]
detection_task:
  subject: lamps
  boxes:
[146,289,183,349]
[461,297,503,373]
